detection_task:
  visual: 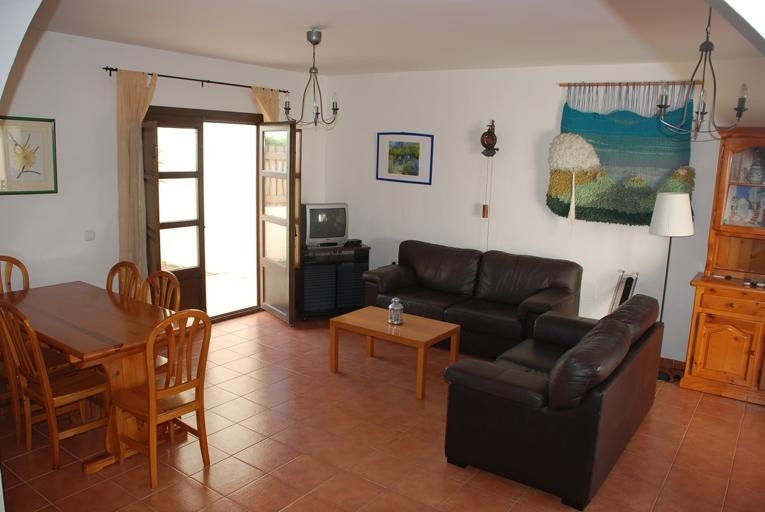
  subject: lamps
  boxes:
[652,5,752,143]
[646,189,696,381]
[281,29,342,133]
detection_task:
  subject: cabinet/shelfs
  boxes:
[678,124,765,407]
[293,241,371,322]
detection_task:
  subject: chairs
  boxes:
[106,260,141,300]
[142,270,180,375]
[113,309,211,489]
[1,255,30,304]
[1,304,109,469]
[1,336,70,446]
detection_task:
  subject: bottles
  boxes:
[387,297,404,325]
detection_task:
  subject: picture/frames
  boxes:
[373,131,436,186]
[0,114,59,196]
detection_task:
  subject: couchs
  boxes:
[359,236,585,363]
[440,289,667,512]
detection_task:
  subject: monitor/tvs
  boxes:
[300,202,349,248]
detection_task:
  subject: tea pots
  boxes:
[742,156,764,186]
